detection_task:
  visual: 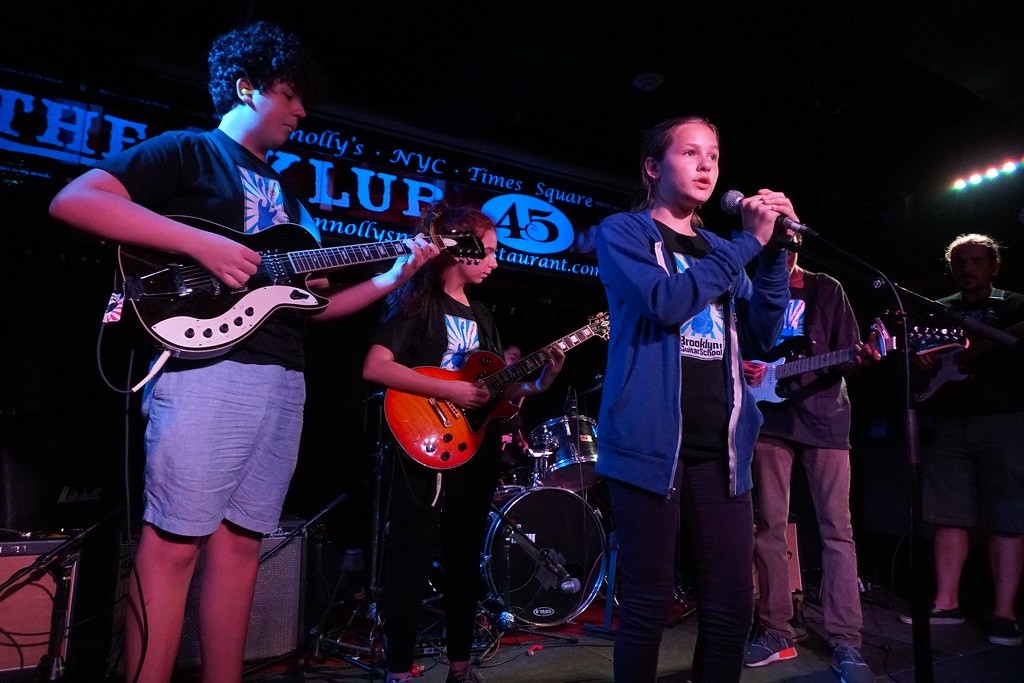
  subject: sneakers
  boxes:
[446,665,487,683]
[385,673,412,683]
[831,644,876,683]
[900,601,966,624]
[987,616,1023,647]
[743,630,798,667]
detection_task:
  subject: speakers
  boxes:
[748,512,804,600]
[0,425,129,535]
[106,518,307,679]
[0,532,81,679]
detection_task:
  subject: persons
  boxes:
[501,340,529,472]
[900,232,1024,646]
[48,21,441,683]
[593,117,801,683]
[745,230,881,683]
[363,203,565,683]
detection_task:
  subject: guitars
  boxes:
[746,324,970,405]
[912,320,1024,402]
[117,212,486,360]
[383,311,611,470]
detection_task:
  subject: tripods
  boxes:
[473,502,579,666]
[331,389,389,655]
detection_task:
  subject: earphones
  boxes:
[241,88,251,95]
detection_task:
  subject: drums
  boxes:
[527,414,602,492]
[483,485,608,627]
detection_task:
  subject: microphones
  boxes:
[554,564,580,594]
[562,388,576,414]
[720,190,818,240]
